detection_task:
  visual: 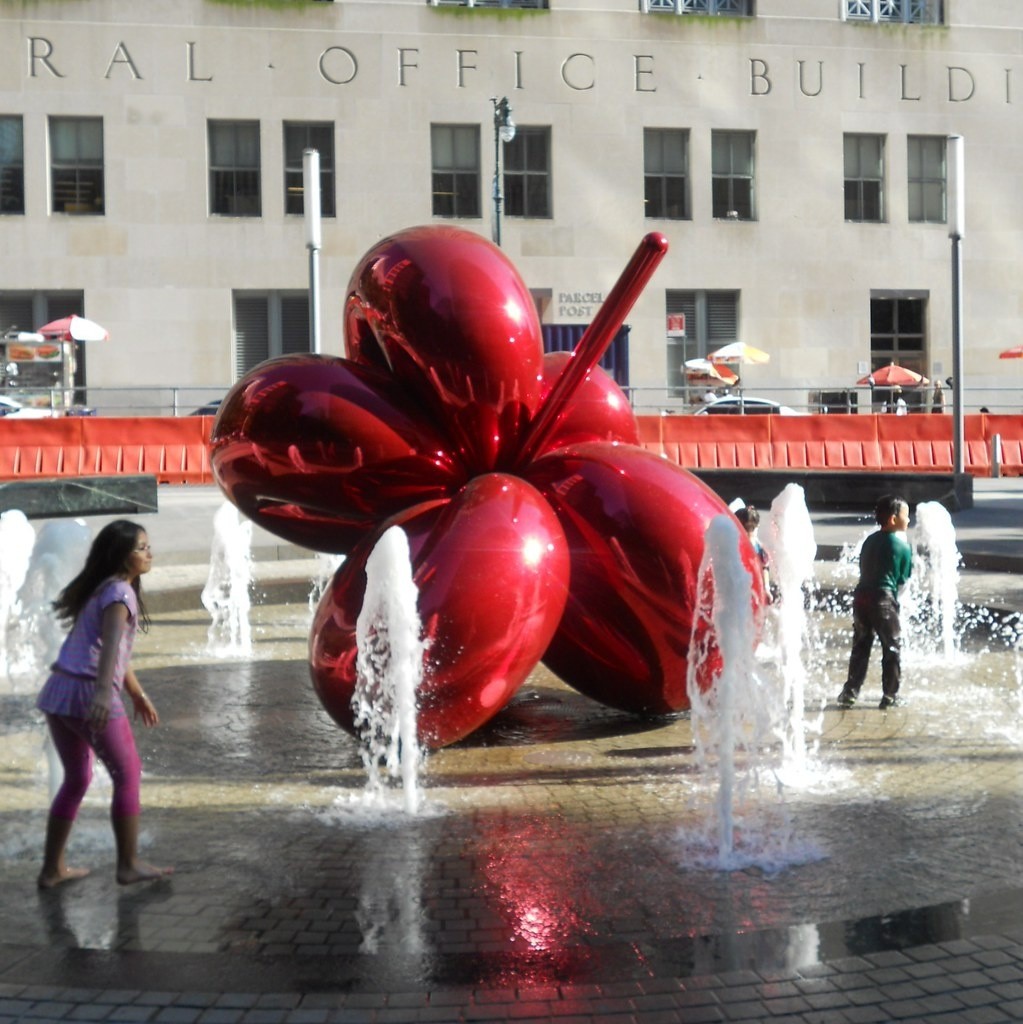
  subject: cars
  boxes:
[693,394,814,417]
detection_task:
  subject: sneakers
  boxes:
[835,688,856,709]
[879,694,906,710]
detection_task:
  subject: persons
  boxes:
[836,497,913,710]
[705,389,717,401]
[36,519,175,888]
[932,380,945,414]
[735,506,774,605]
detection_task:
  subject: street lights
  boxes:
[487,95,517,247]
[946,134,966,472]
[302,147,322,353]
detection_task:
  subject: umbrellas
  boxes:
[855,360,929,413]
[37,314,109,385]
[680,359,739,387]
[999,345,1023,359]
[705,343,770,387]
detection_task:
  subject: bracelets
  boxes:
[133,693,147,702]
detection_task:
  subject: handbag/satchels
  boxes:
[764,579,783,605]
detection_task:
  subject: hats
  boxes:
[734,504,760,529]
